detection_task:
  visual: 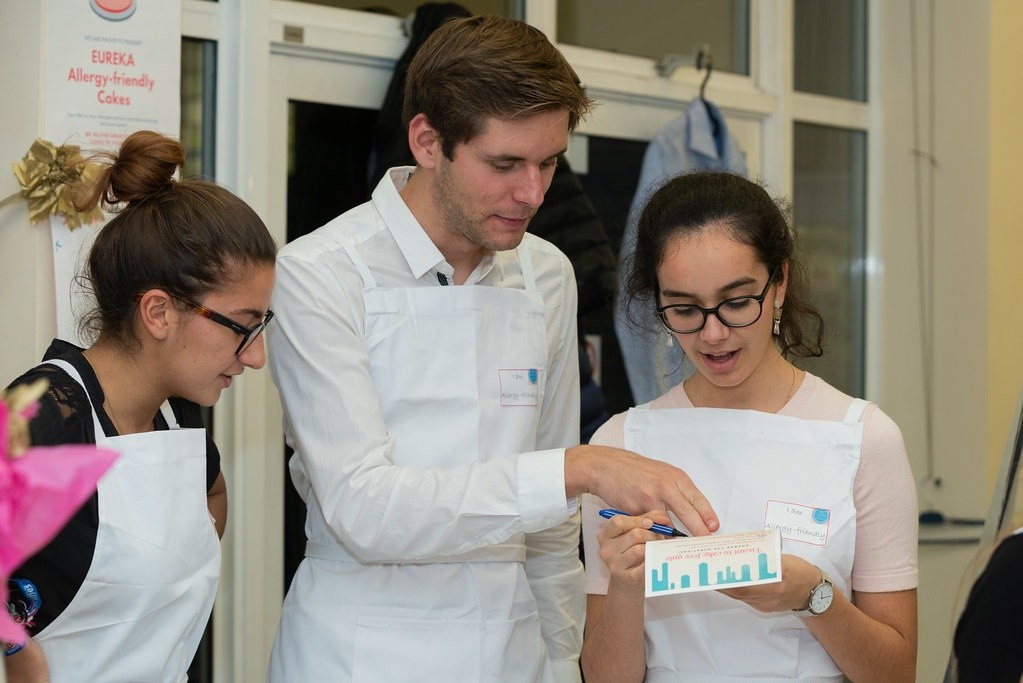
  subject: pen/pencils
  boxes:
[599,510,689,538]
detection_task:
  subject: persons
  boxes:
[575,170,921,683]
[250,14,723,683]
[0,128,282,683]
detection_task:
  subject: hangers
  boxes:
[696,49,722,140]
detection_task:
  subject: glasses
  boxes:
[139,283,276,359]
[653,263,781,335]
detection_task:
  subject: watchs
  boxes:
[793,562,837,622]
